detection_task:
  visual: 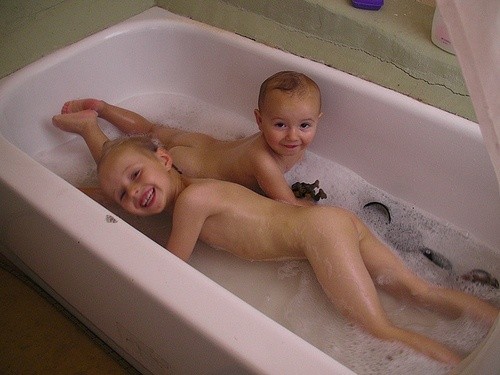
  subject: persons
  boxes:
[51,71,326,209]
[75,135,499,370]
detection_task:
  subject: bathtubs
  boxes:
[0,6,499,375]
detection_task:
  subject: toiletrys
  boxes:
[431,5,459,58]
